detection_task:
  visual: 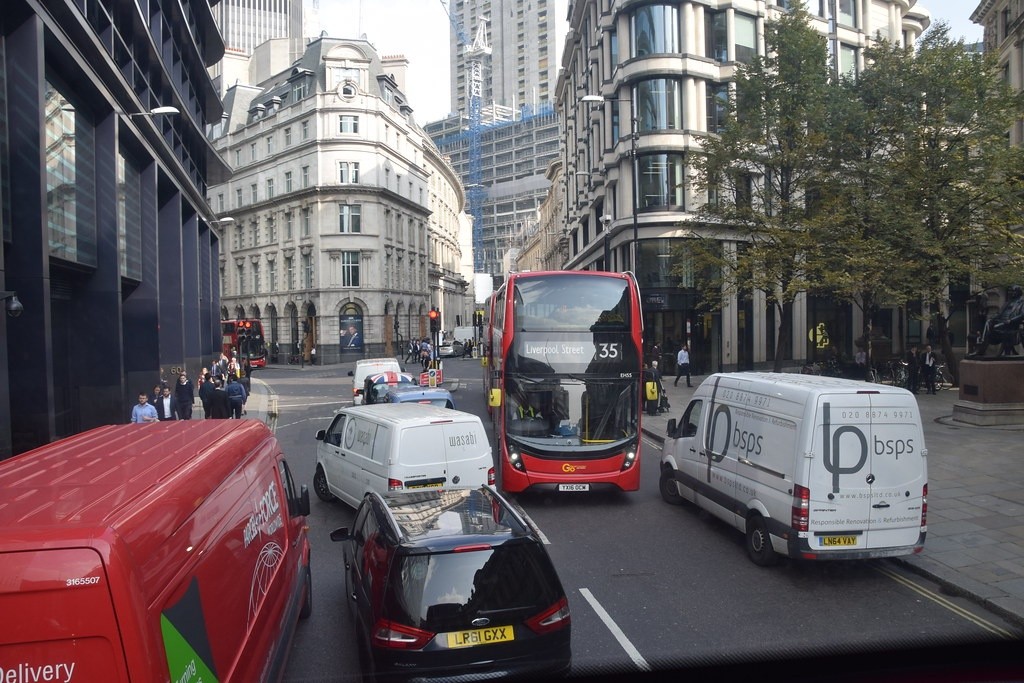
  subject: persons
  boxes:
[970,284,1024,355]
[405,336,475,373]
[832,322,981,393]
[641,340,667,415]
[310,346,317,365]
[512,395,543,420]
[347,326,361,347]
[131,347,252,424]
[674,344,693,387]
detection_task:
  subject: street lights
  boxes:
[582,95,639,277]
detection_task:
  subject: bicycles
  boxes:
[871,361,955,391]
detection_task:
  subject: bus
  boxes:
[482,270,657,495]
[221,319,266,371]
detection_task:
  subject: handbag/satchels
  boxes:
[233,374,238,381]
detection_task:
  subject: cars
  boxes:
[439,341,464,357]
[356,373,417,405]
[383,387,455,409]
[330,484,572,683]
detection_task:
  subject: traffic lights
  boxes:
[429,311,441,332]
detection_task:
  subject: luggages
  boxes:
[659,389,670,413]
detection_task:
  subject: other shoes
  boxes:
[674,382,677,387]
[688,385,692,387]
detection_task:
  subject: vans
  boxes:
[312,402,497,510]
[658,375,929,567]
[0,419,312,683]
[347,358,407,406]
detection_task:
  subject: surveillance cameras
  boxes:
[600,215,611,222]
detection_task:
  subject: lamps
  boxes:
[129,105,180,119]
[210,217,235,224]
[576,171,606,177]
[581,94,619,103]
